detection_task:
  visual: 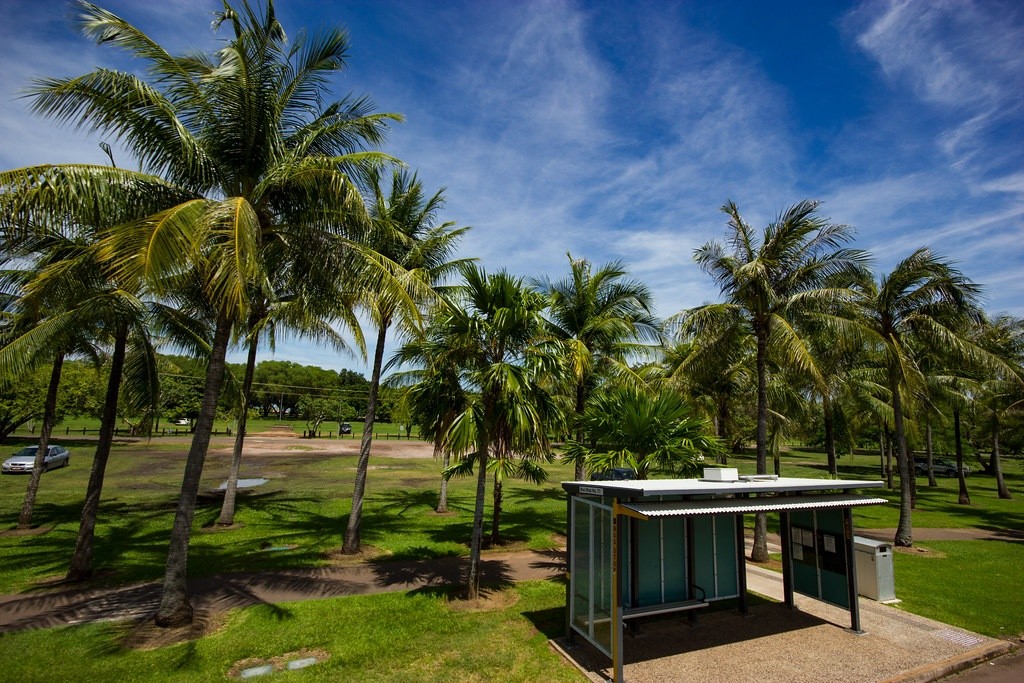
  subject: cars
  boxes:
[340,422,351,434]
[175,417,191,426]
[3,443,70,475]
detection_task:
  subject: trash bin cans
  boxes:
[854,536,896,602]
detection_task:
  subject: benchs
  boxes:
[576,584,709,641]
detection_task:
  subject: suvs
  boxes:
[906,456,969,478]
